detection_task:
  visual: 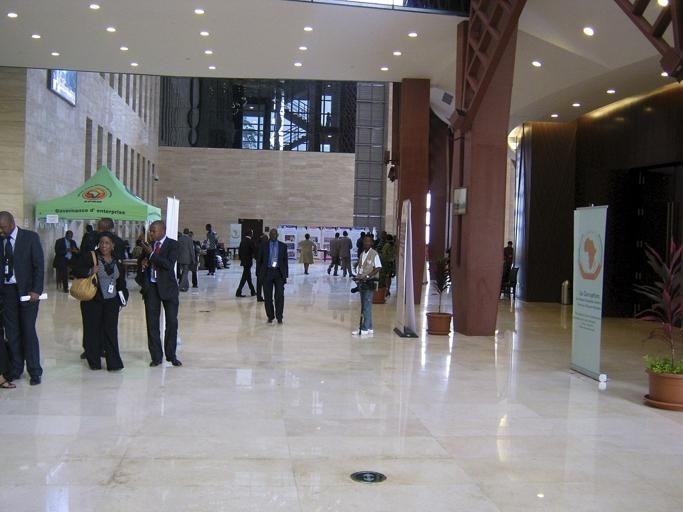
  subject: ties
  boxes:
[153,242,159,278]
[5,238,13,281]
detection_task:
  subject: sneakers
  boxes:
[352,329,373,335]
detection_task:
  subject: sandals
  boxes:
[0,380,16,388]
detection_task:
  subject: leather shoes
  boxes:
[150,360,181,367]
[30,376,41,385]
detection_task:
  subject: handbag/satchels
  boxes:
[70,277,97,301]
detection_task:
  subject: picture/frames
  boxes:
[451,185,466,217]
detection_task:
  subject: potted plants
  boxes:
[423,245,452,336]
[374,252,395,306]
[631,235,683,411]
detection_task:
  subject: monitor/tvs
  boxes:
[48,70,77,107]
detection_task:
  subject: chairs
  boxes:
[500,264,518,303]
[51,257,139,289]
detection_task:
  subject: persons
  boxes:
[0,211,43,385]
[300,235,317,275]
[136,222,232,368]
[0,312,17,389]
[237,228,288,323]
[326,231,395,336]
[504,241,513,268]
[54,220,128,372]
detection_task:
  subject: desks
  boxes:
[296,248,327,262]
[227,246,240,260]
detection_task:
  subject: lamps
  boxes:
[383,158,399,183]
[152,171,159,183]
